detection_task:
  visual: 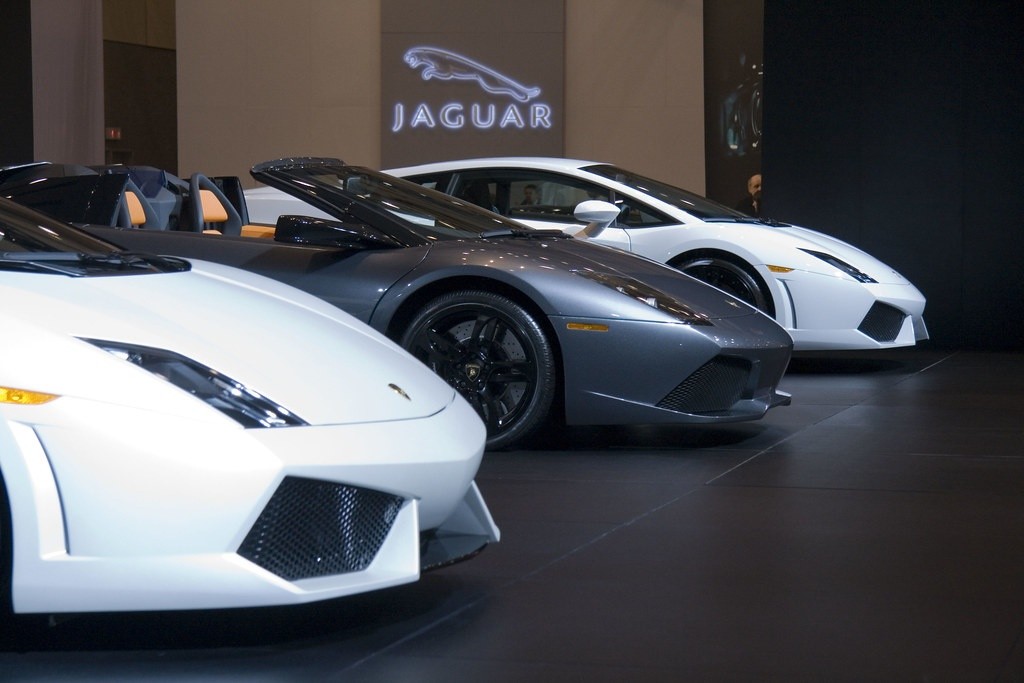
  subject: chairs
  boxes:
[189,173,242,238]
[109,176,162,231]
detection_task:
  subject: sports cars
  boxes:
[0,158,795,451]
[244,156,928,364]
[0,199,500,624]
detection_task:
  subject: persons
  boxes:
[745,174,761,218]
[587,190,609,203]
[520,184,546,205]
